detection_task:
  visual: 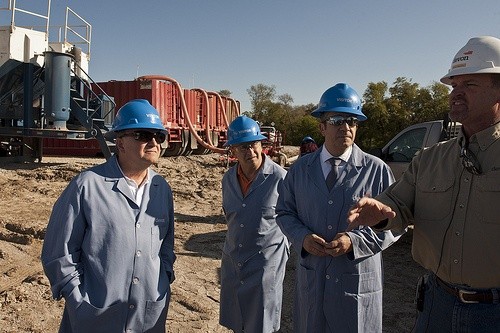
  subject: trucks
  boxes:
[375,120,463,181]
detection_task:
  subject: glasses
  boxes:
[233,141,260,151]
[118,129,166,143]
[323,114,359,127]
[457,137,481,176]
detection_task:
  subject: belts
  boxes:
[430,273,500,306]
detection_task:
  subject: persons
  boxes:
[276,84,408,333]
[220,115,289,333]
[348,37,500,333]
[262,144,291,165]
[301,136,318,156]
[41,98,177,333]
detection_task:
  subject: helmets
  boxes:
[439,35,500,85]
[310,83,368,122]
[222,114,268,146]
[300,136,316,145]
[104,99,169,143]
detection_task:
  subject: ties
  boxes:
[325,158,343,192]
